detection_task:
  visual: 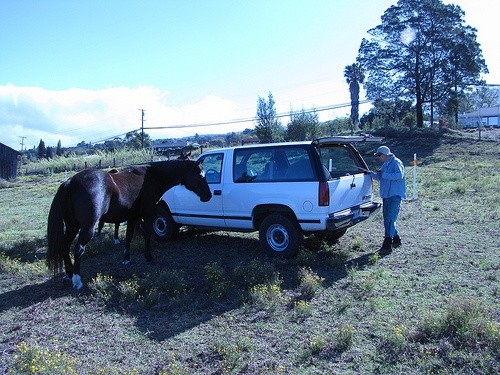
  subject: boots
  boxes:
[392,235,401,247]
[375,237,392,255]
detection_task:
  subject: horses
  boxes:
[46,151,212,293]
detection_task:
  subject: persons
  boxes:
[363,145,408,255]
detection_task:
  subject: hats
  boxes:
[374,146,393,156]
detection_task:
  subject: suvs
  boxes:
[152,136,385,258]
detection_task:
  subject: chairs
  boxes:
[236,163,250,182]
[264,161,279,179]
